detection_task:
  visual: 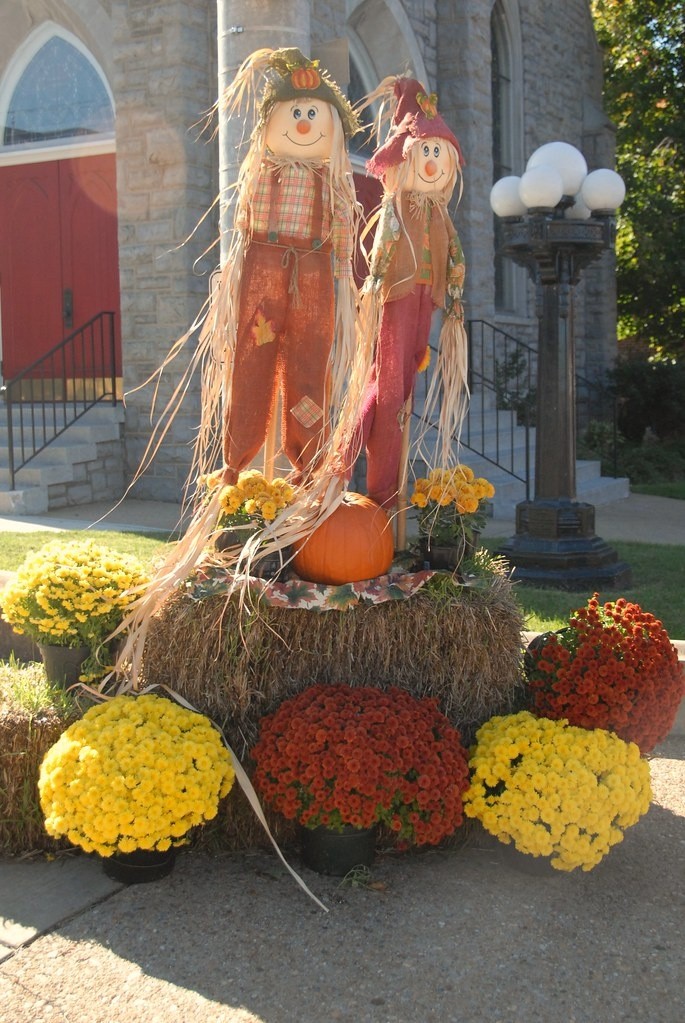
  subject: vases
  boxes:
[234,550,290,583]
[34,640,90,697]
[524,631,556,682]
[417,534,480,570]
[498,832,556,878]
[290,816,383,878]
[101,832,176,885]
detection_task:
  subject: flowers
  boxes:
[35,694,235,858]
[531,592,685,756]
[246,685,471,855]
[461,712,653,872]
[411,463,495,548]
[0,540,155,690]
[197,470,297,556]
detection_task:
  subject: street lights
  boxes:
[489,139,627,589]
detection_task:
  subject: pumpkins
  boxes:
[292,489,394,585]
